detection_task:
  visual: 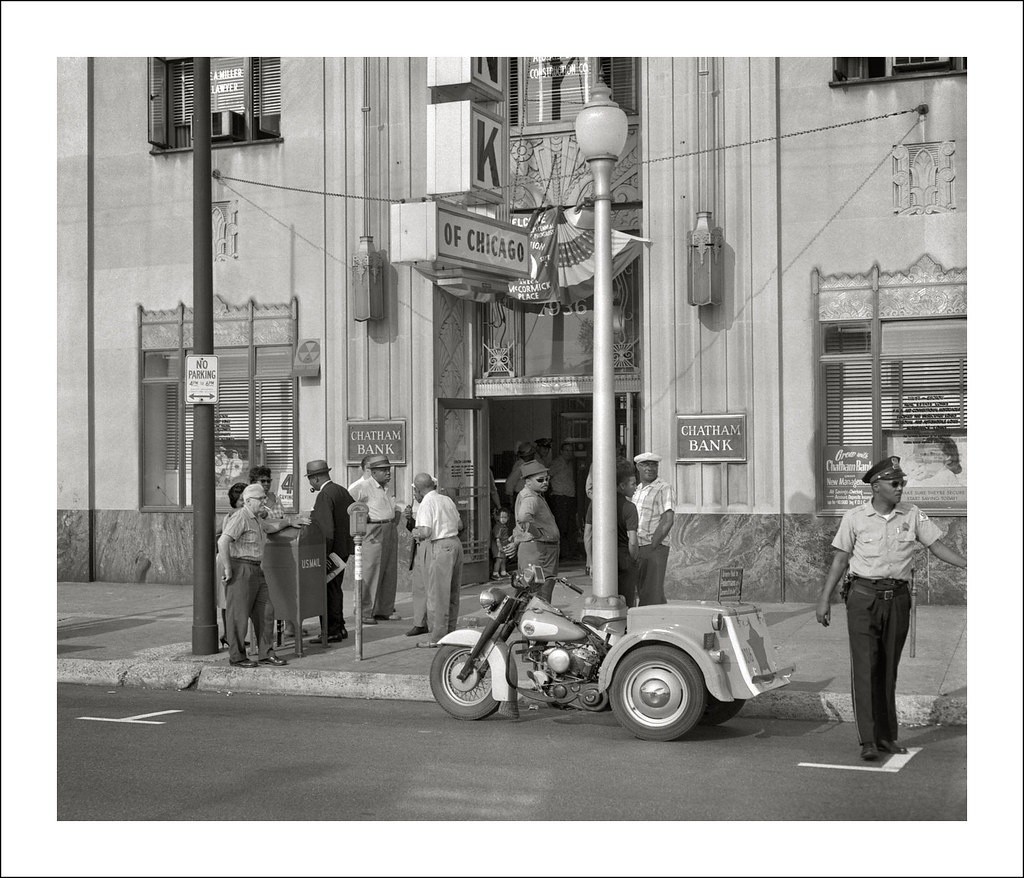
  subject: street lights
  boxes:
[572,67,629,629]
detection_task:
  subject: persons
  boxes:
[216,482,251,649]
[347,454,401,623]
[217,484,311,668]
[304,460,356,644]
[412,473,464,648]
[903,437,963,487]
[502,462,560,663]
[816,455,967,758]
[215,447,243,489]
[489,468,501,510]
[583,460,640,608]
[505,438,626,560]
[490,508,512,580]
[249,465,285,522]
[630,452,675,605]
[404,477,438,636]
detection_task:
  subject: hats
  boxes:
[633,452,662,463]
[534,437,553,448]
[520,460,550,479]
[862,456,907,484]
[366,456,393,469]
[303,460,331,476]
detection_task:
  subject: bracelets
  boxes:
[536,534,542,539]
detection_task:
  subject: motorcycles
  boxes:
[429,557,795,743]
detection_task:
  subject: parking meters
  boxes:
[345,501,370,661]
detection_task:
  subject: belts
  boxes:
[852,584,908,600]
[368,519,395,523]
[530,540,558,544]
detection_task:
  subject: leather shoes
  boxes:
[876,738,907,755]
[861,742,878,758]
[258,656,287,666]
[230,660,258,667]
[309,632,342,643]
[342,629,348,639]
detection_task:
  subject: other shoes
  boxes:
[375,613,401,620]
[361,616,377,624]
[500,572,512,579]
[406,626,429,637]
[491,573,504,581]
[416,639,437,648]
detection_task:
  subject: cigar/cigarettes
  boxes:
[263,505,270,510]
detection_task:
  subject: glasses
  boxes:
[256,479,271,484]
[250,496,266,501]
[879,480,907,487]
[530,477,548,482]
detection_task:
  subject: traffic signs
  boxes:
[183,355,219,405]
